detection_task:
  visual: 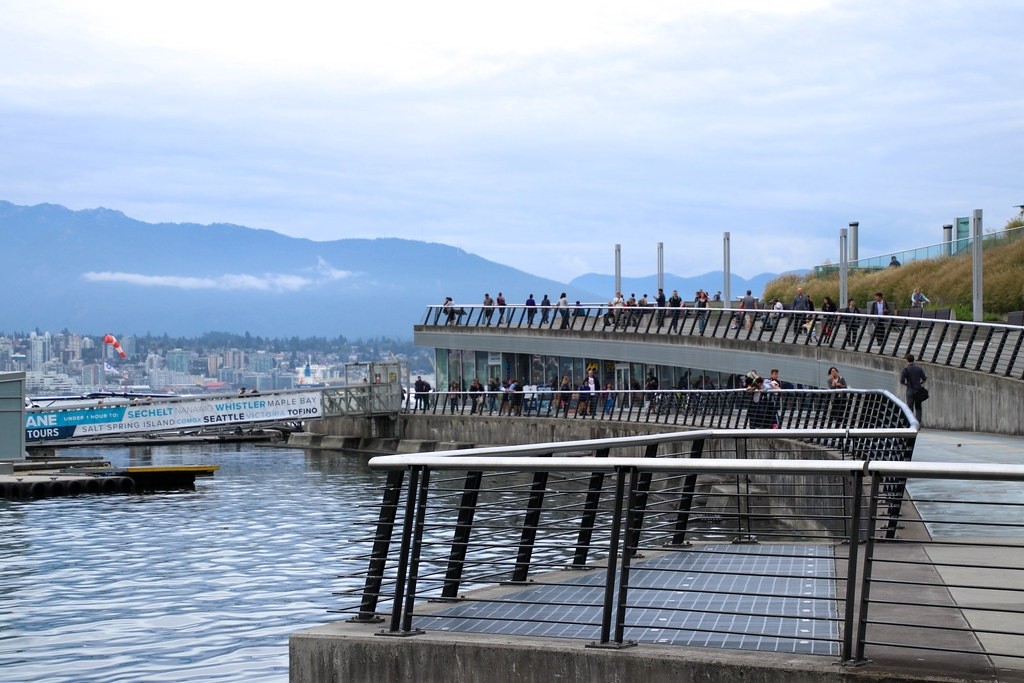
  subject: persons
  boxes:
[90,376,370,410]
[393,282,933,428]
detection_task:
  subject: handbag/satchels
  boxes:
[478,395,483,404]
[912,386,929,402]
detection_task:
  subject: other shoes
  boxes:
[448,319,886,347]
[414,406,655,417]
